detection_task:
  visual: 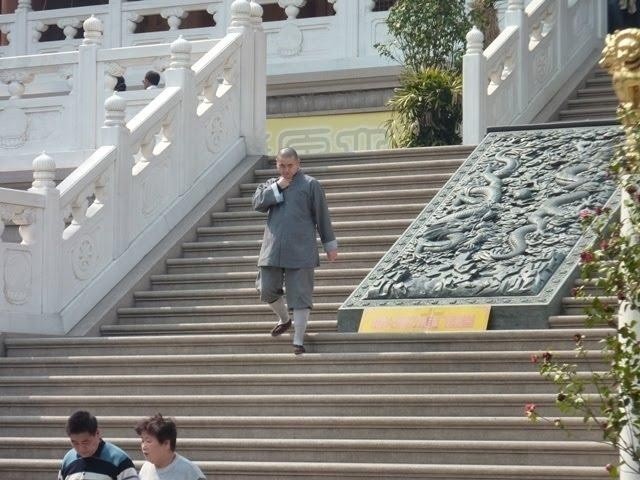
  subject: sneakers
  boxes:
[294,345,304,354]
[271,319,291,336]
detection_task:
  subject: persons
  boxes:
[134,412,207,480]
[250,147,339,354]
[58,411,138,480]
